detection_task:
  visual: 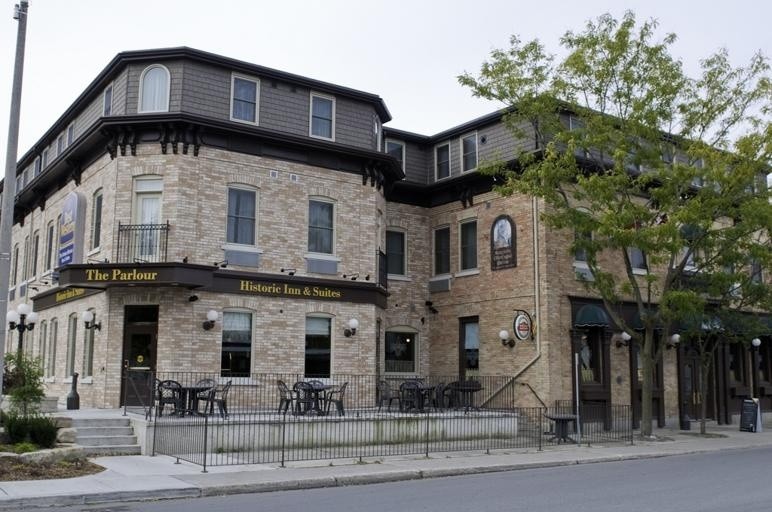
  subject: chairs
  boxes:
[159,378,232,419]
[277,379,348,418]
[378,379,483,415]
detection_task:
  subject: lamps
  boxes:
[666,333,680,350]
[499,331,515,347]
[27,274,60,292]
[343,272,360,280]
[750,338,761,351]
[133,257,151,269]
[281,267,296,276]
[214,260,229,267]
[616,331,631,348]
[82,311,101,331]
[203,310,220,330]
[87,258,108,268]
[344,318,358,336]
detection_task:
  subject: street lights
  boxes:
[6,302,40,372]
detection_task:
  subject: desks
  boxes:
[546,413,578,444]
